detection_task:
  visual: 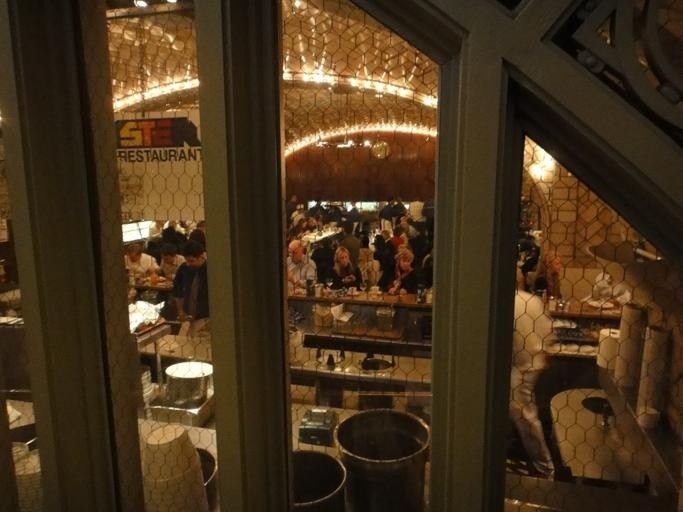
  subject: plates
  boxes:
[346,291,360,296]
[139,363,157,422]
[587,299,614,308]
[8,441,48,512]
[550,342,599,355]
[368,291,383,295]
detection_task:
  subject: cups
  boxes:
[348,286,357,294]
[326,277,334,287]
[370,286,380,292]
[150,269,159,283]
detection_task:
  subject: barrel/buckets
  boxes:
[290,450,346,512]
[142,423,216,512]
[165,362,214,410]
[140,364,155,421]
[332,408,429,511]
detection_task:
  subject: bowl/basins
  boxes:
[142,424,210,512]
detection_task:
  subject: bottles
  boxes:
[375,306,394,332]
[416,282,426,303]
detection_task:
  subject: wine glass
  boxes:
[359,283,368,296]
